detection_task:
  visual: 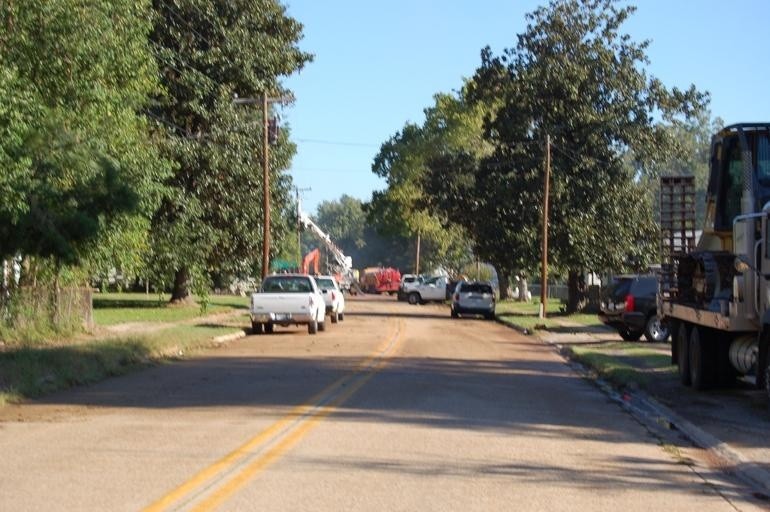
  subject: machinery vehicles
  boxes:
[361,267,400,292]
[657,120,770,414]
[303,219,359,295]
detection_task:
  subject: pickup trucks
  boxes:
[249,273,326,333]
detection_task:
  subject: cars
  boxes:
[401,273,449,304]
[314,275,346,323]
[450,280,496,319]
[599,275,670,342]
